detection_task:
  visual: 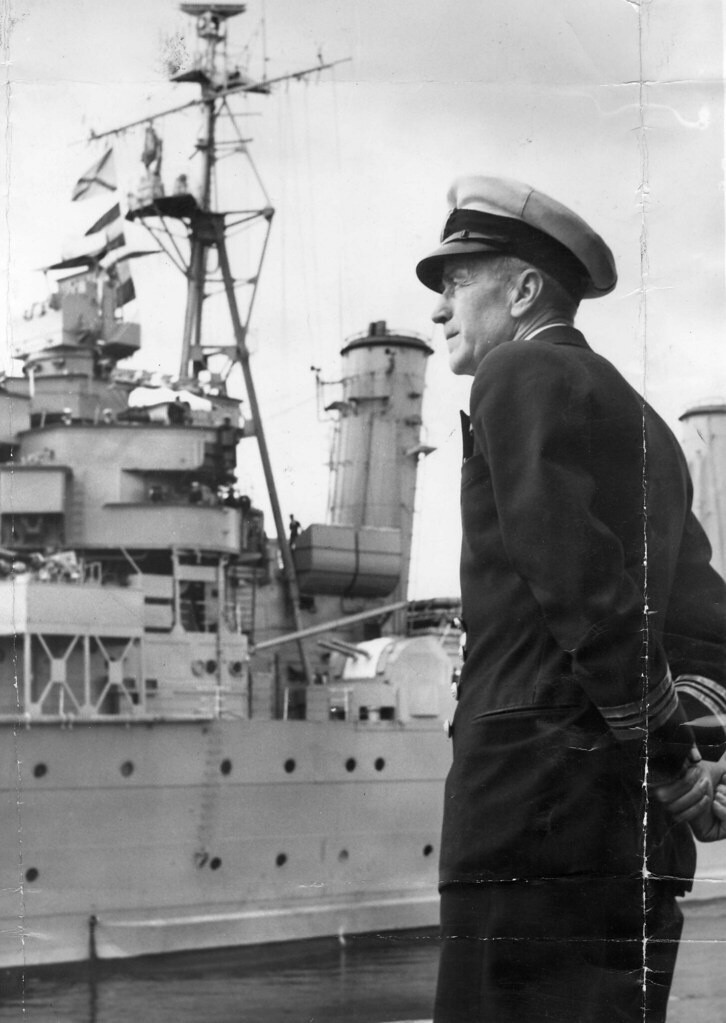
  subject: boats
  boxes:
[0,0,725,967]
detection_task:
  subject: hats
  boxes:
[416,176,618,308]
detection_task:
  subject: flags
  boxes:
[116,261,136,307]
[72,149,117,202]
[86,204,125,254]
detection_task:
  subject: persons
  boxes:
[226,488,236,503]
[149,487,162,502]
[289,514,303,539]
[415,176,726,1023]
[189,481,203,504]
